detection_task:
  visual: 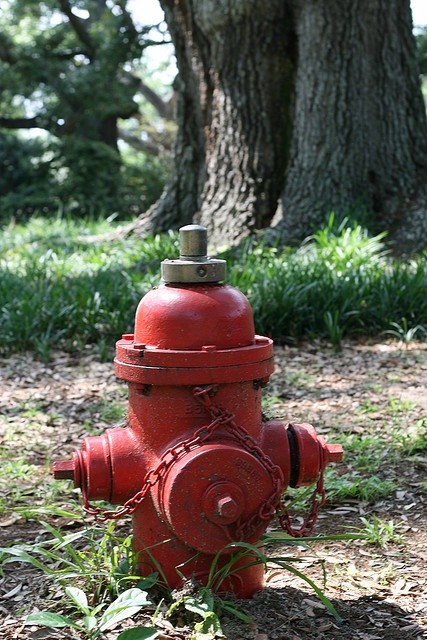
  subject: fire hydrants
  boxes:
[52,223,344,599]
